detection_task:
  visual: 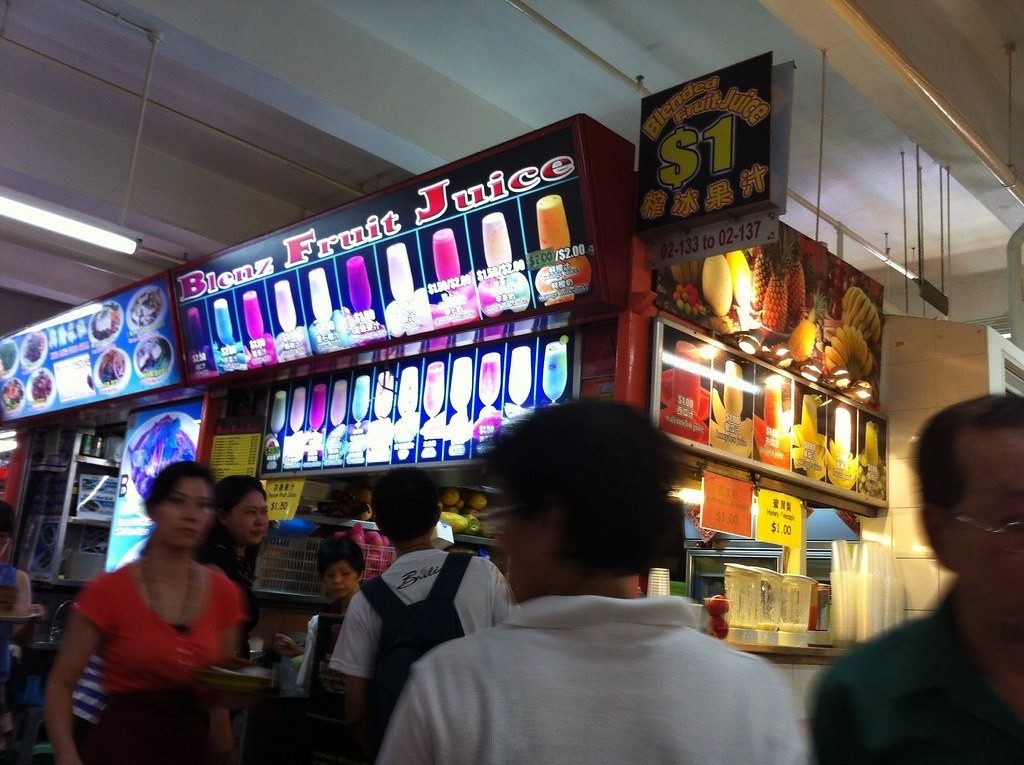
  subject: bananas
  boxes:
[822,286,881,383]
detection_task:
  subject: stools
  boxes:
[9,705,45,765]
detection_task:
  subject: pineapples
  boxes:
[749,224,827,362]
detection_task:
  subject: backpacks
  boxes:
[360,552,471,738]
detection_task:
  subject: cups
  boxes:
[647,567,671,597]
[701,598,734,643]
[829,539,905,649]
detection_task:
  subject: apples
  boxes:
[705,595,731,639]
[331,524,395,580]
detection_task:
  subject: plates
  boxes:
[185,667,285,697]
[134,334,175,381]
[88,300,124,346]
[26,367,56,409]
[93,348,132,393]
[122,411,200,523]
[0,609,41,623]
[126,285,167,333]
[0,378,26,413]
[20,331,48,370]
[0,340,19,380]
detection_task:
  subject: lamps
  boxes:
[759,341,794,368]
[721,328,765,354]
[792,357,825,382]
[819,365,851,387]
[842,380,874,404]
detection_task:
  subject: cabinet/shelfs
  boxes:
[251,513,500,606]
[11,427,121,589]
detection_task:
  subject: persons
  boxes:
[809,393,1024,765]
[43,462,270,765]
[0,502,44,755]
[377,396,808,765]
[275,462,513,753]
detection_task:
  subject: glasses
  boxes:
[0,534,13,544]
[940,505,1024,552]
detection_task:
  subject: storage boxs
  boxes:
[252,534,326,598]
[356,543,396,584]
